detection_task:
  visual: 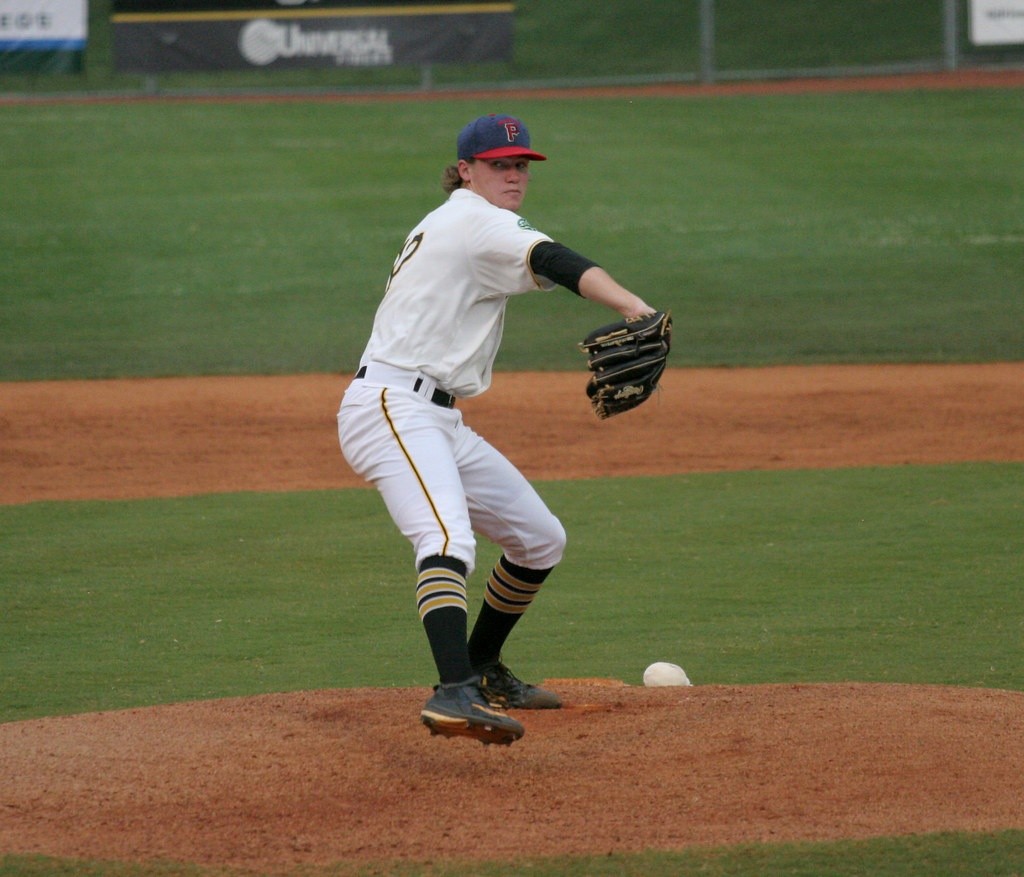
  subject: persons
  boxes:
[333,110,673,747]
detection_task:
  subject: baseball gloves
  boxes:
[580,303,673,422]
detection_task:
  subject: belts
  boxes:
[354,366,456,409]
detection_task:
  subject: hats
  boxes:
[456,113,547,161]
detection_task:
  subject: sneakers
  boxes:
[421,677,524,747]
[466,644,562,709]
